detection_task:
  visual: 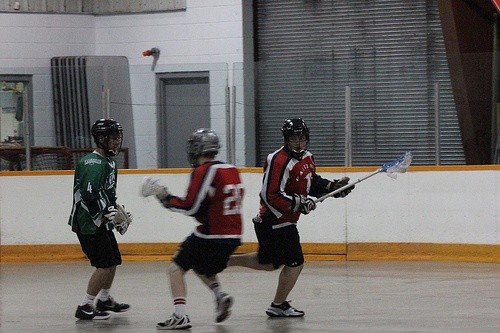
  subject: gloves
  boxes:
[115,210,134,235]
[101,203,118,228]
[157,186,172,207]
[326,177,355,198]
[291,193,317,216]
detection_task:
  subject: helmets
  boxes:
[91,118,123,157]
[280,117,311,161]
[185,128,219,169]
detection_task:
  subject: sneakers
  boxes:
[214,293,233,323]
[156,313,191,329]
[75,303,111,320]
[95,297,130,312]
[265,301,305,318]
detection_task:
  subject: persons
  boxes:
[140,126,245,330]
[66,117,131,321]
[225,119,356,318]
